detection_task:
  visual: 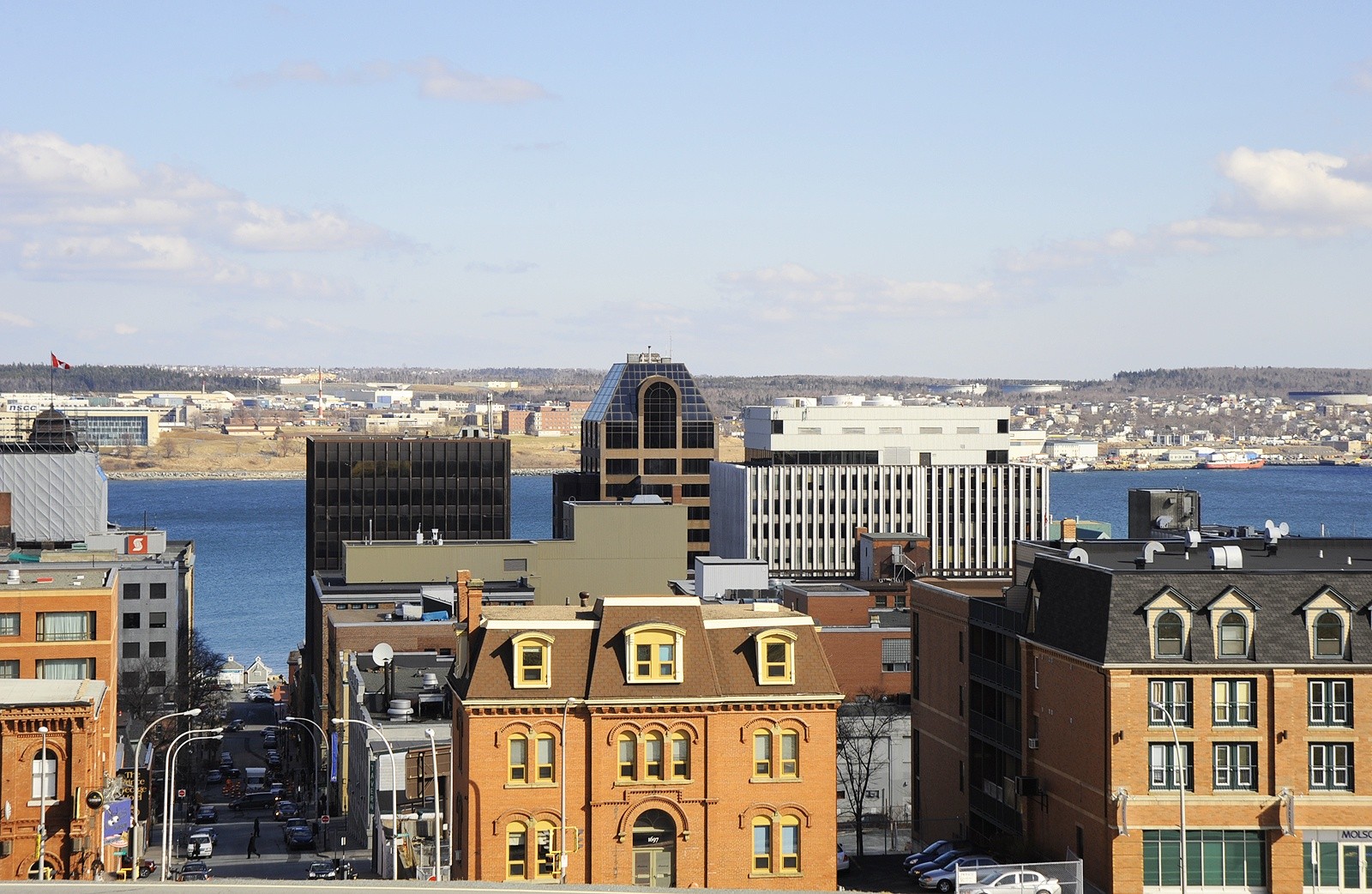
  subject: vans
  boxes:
[228,793,276,810]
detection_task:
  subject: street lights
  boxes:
[37,726,48,886]
[423,728,442,882]
[331,717,398,882]
[161,728,224,881]
[168,735,222,870]
[134,708,202,884]
[277,716,329,848]
[1149,700,1187,894]
[561,696,576,884]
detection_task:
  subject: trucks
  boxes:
[241,766,268,796]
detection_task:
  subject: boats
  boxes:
[1196,448,1264,469]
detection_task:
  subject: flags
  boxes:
[52,354,70,369]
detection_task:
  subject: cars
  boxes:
[227,719,246,732]
[905,839,1062,894]
[309,863,335,880]
[836,842,850,869]
[276,801,313,845]
[245,683,274,704]
[260,726,285,798]
[206,752,242,784]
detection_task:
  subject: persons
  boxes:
[301,769,306,782]
[191,839,201,861]
[306,790,313,804]
[247,833,260,859]
[254,817,261,837]
[320,793,327,814]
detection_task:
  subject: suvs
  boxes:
[179,861,210,880]
[121,856,157,877]
[186,825,217,855]
[196,806,217,823]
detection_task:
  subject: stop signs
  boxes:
[322,816,330,823]
[178,790,186,798]
